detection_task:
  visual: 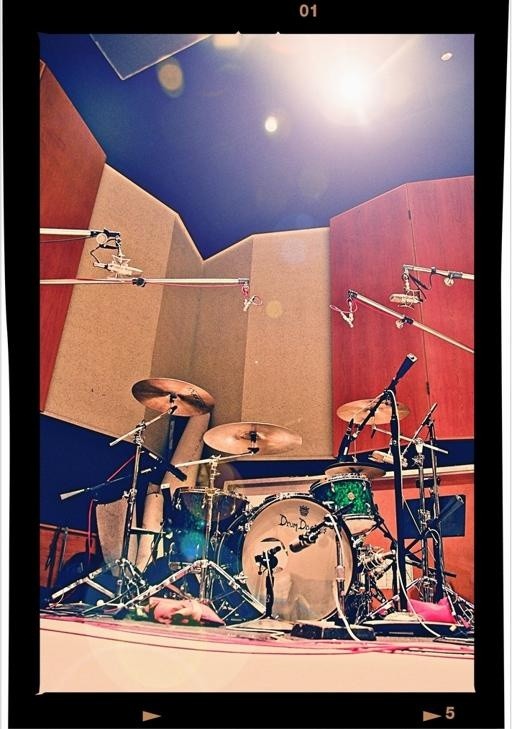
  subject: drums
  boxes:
[168,485,249,571]
[213,496,358,622]
[309,474,379,534]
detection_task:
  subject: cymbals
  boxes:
[202,422,302,456]
[326,462,386,481]
[132,377,214,418]
[336,398,406,427]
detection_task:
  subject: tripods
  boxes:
[356,424,475,626]
[140,447,281,625]
[51,404,152,613]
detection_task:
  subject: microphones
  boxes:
[370,450,408,468]
[289,530,322,553]
[336,417,355,463]
[92,262,143,278]
[389,293,423,306]
